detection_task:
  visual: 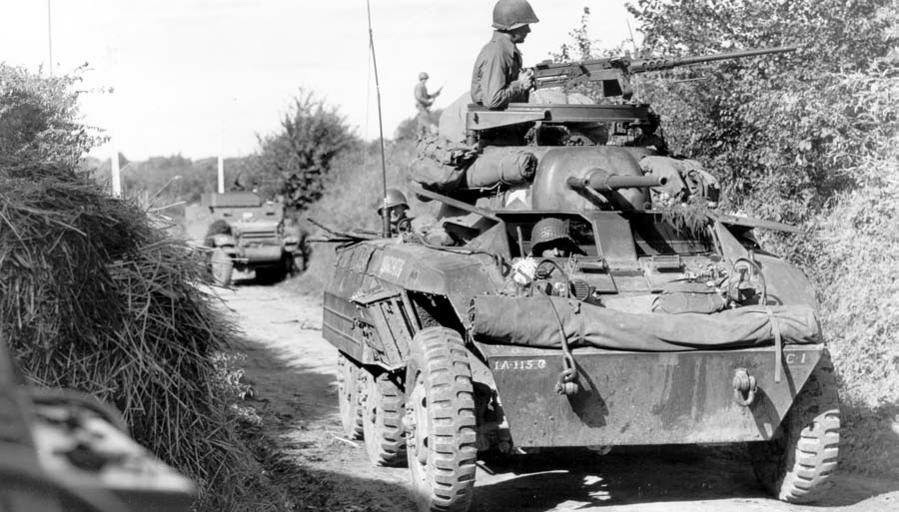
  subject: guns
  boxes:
[522,46,799,97]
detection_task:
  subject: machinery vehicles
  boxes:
[1,325,200,511]
[184,190,305,288]
[304,44,841,511]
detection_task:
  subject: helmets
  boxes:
[490,0,539,30]
[376,189,410,216]
[419,72,429,81]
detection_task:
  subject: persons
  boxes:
[470,0,538,110]
[373,188,410,231]
[413,71,435,138]
